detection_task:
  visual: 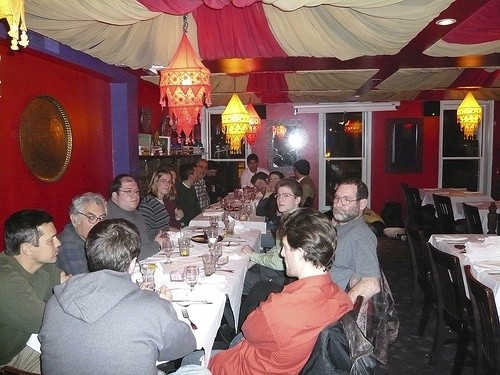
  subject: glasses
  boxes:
[77,211,106,224]
[274,193,297,199]
[330,195,363,206]
[116,188,143,196]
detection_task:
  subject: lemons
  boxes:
[182,250,187,255]
[148,264,157,270]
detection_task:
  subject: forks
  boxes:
[182,309,197,330]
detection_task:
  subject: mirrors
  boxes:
[385,117,424,174]
[265,118,303,175]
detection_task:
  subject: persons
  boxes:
[176,160,225,228]
[212,159,381,350]
[56,192,107,276]
[181,207,353,375]
[38,218,197,375]
[106,174,171,261]
[137,166,184,235]
[0,209,72,374]
[240,154,270,188]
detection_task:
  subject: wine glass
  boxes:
[209,244,222,268]
[162,240,174,264]
[185,265,199,292]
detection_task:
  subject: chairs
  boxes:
[302,195,318,208]
[300,295,374,375]
[399,182,500,375]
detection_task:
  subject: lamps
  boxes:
[456,90,483,140]
[158,14,213,145]
[245,97,261,149]
[343,112,362,140]
[221,73,249,155]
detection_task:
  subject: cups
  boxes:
[207,230,218,251]
[202,254,215,276]
[143,270,155,290]
[220,186,257,221]
[223,213,236,237]
[178,237,190,256]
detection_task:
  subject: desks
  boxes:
[188,189,268,235]
[434,191,493,222]
[464,201,500,234]
[417,186,467,204]
[27,226,261,375]
[428,232,500,318]
